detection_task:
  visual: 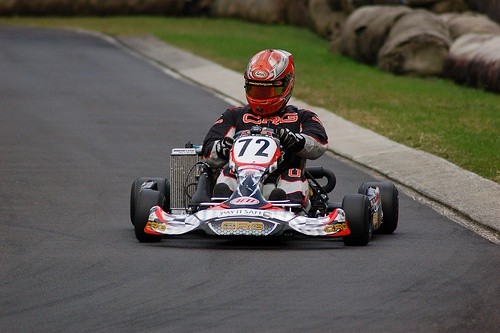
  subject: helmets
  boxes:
[244,49,295,115]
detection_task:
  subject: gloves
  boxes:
[272,127,305,153]
[216,137,233,160]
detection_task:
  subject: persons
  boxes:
[200,48,329,203]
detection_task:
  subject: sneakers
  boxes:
[213,183,231,197]
[269,188,286,201]
